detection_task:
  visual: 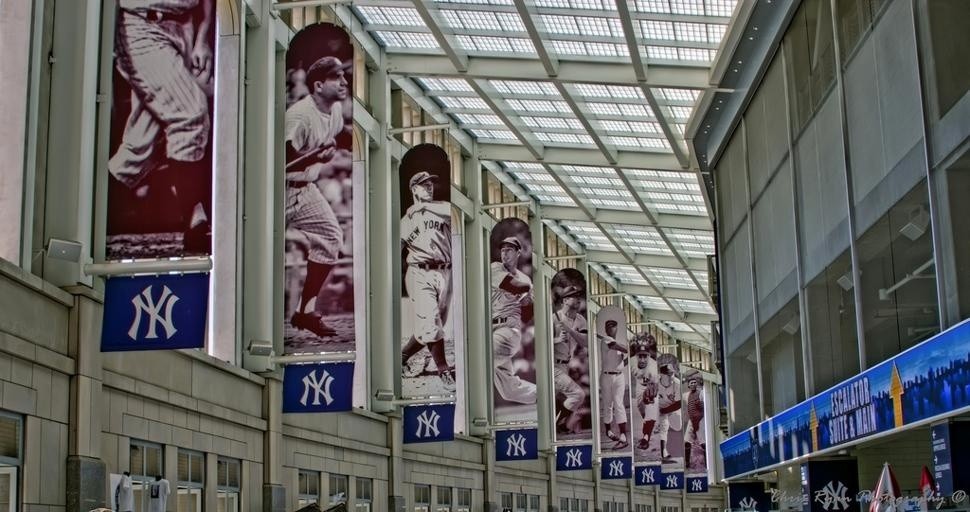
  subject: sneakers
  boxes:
[291,309,338,338]
[606,430,619,441]
[612,441,629,449]
[637,439,649,450]
[439,369,456,391]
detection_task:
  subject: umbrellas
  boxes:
[869,462,903,511]
[918,464,935,509]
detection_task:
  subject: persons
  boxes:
[597,319,631,449]
[627,344,661,446]
[400,171,454,385]
[659,363,683,462]
[107,0,212,238]
[284,53,353,337]
[682,376,707,467]
[490,237,537,406]
[551,287,587,435]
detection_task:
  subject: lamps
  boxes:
[470,417,487,427]
[372,389,393,401]
[244,338,273,357]
[29,236,86,270]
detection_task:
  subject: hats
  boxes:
[605,320,618,329]
[409,172,437,188]
[659,363,675,375]
[496,237,521,251]
[562,285,584,297]
[636,344,650,356]
[303,56,352,85]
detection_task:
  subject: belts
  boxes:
[491,318,507,324]
[285,179,308,189]
[417,262,452,270]
[554,359,570,364]
[604,371,622,374]
